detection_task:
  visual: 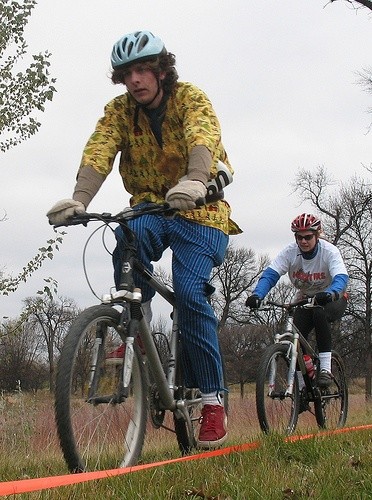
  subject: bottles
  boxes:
[304,354,315,379]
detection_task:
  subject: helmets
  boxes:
[290,212,322,239]
[109,30,166,69]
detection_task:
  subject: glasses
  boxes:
[294,233,316,240]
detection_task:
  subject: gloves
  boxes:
[46,199,86,225]
[315,291,332,306]
[245,294,262,308]
[170,197,197,212]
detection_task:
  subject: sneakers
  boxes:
[196,404,228,448]
[297,395,307,414]
[104,343,127,365]
[318,371,332,387]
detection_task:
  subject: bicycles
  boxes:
[248,292,349,440]
[53,196,230,474]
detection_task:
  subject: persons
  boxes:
[46,30,243,448]
[246,213,349,415]
